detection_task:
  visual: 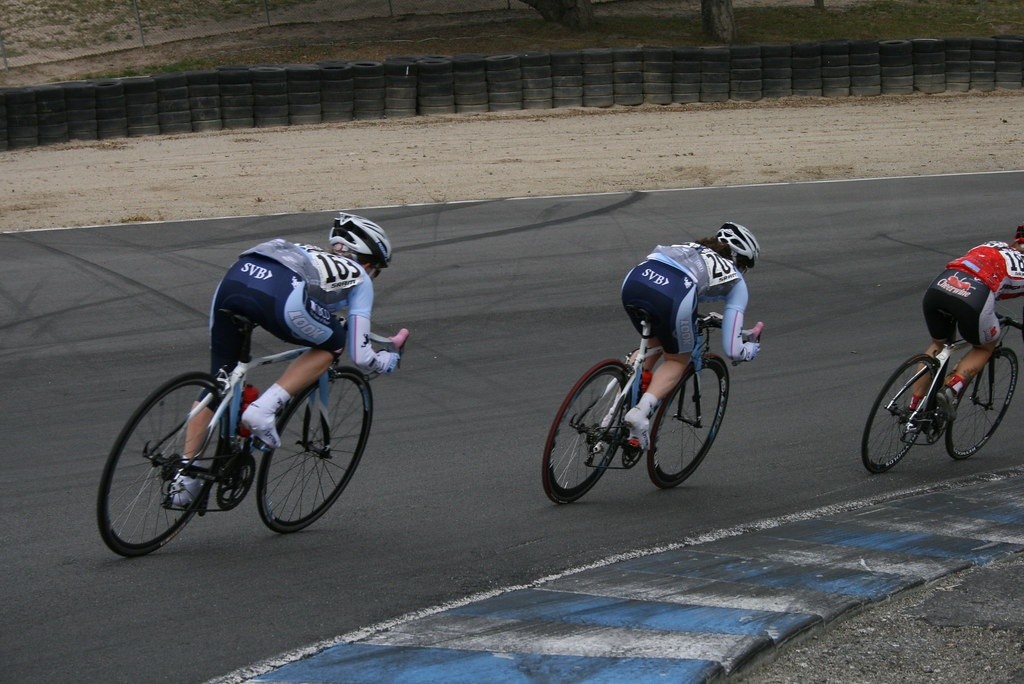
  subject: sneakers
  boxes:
[624,408,650,450]
[165,478,202,510]
[936,385,959,421]
[602,413,621,435]
[905,406,923,419]
[244,404,281,447]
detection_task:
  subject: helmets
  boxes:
[329,212,391,267]
[716,221,760,268]
[1014,225,1024,244]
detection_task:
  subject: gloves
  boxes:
[742,342,761,361]
[374,349,401,375]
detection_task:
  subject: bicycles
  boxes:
[97,327,411,557]
[540,312,764,504]
[862,313,1024,476]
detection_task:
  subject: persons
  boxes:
[600,221,761,450]
[907,224,1024,418]
[169,212,400,510]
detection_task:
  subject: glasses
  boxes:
[739,263,748,274]
[370,263,381,277]
